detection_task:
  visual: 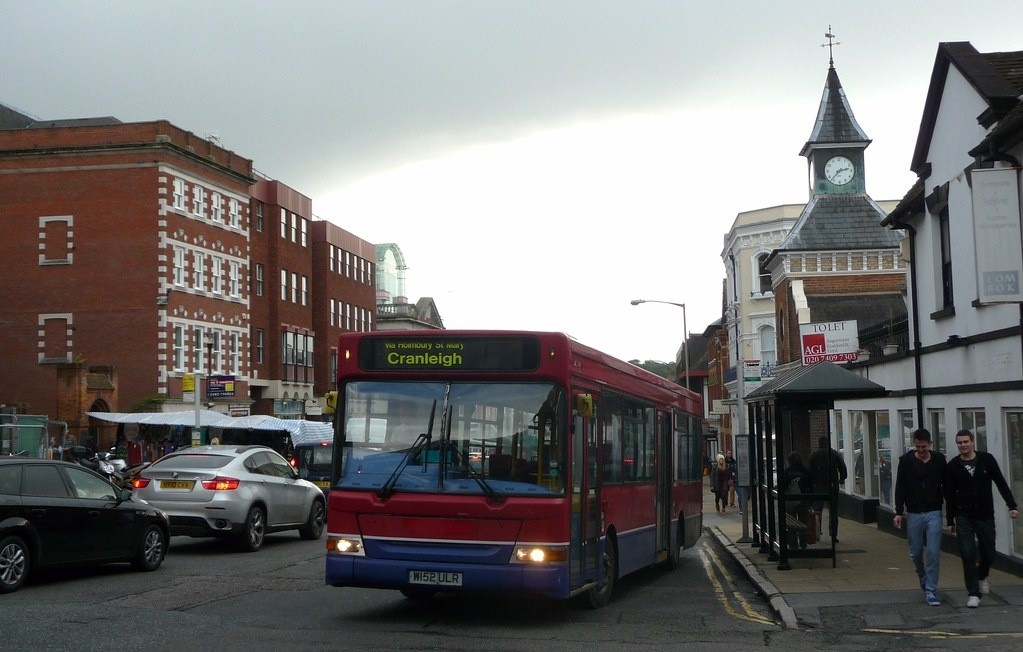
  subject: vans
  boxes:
[291,440,385,501]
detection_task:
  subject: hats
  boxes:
[716,454,724,461]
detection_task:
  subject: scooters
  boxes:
[69,445,124,488]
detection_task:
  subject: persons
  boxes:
[63,430,77,462]
[111,438,128,460]
[164,440,174,455]
[784,451,813,549]
[945,430,1020,607]
[893,429,948,605]
[382,400,425,451]
[855,445,865,495]
[879,452,892,505]
[807,436,847,543]
[709,450,743,516]
[50,437,61,460]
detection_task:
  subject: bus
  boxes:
[324,330,704,612]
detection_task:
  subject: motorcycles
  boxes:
[98,447,152,483]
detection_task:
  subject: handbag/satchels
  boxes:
[785,482,802,510]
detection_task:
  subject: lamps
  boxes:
[947,335,960,344]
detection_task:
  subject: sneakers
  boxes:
[926,589,940,605]
[968,596,980,607]
[979,579,989,595]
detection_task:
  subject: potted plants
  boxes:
[883,310,899,355]
[857,349,871,361]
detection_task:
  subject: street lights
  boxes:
[630,298,690,390]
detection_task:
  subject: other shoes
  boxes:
[716,506,720,514]
[830,536,839,543]
[722,509,727,516]
[730,504,736,508]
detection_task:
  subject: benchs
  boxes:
[786,514,809,550]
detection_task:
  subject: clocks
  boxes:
[824,155,856,186]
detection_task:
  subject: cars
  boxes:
[0,455,171,595]
[469,451,489,459]
[131,444,328,553]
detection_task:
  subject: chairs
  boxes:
[491,438,567,488]
